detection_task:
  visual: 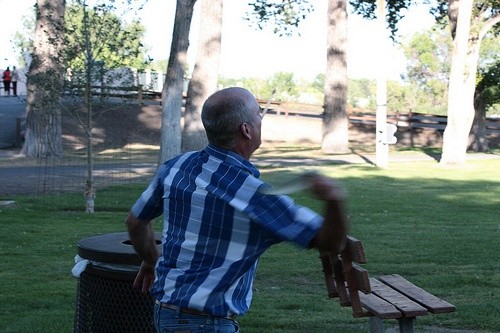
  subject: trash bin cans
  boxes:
[71,231,163,333]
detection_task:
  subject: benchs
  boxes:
[320,233,456,333]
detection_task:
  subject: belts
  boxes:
[154,299,238,321]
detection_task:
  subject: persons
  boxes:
[125,87,347,333]
[3,66,18,96]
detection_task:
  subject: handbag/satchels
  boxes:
[2,72,6,78]
